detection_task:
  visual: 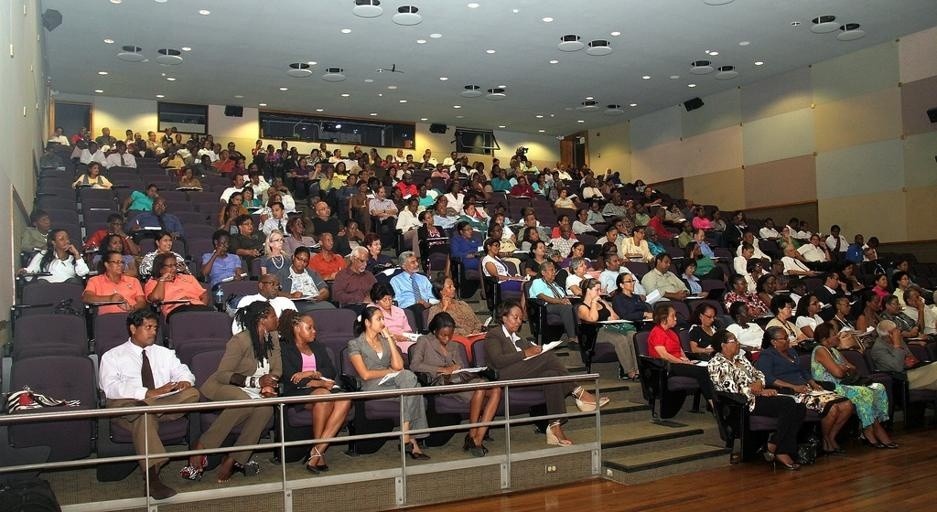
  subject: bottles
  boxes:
[216,284,224,304]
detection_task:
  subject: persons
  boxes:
[18,126,937,468]
[96,306,200,502]
[181,302,284,484]
[275,307,353,475]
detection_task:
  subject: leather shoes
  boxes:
[145,481,177,500]
[819,431,848,456]
[863,429,901,448]
[480,290,487,301]
[118,404,140,422]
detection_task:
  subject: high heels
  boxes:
[755,437,800,471]
[302,446,329,474]
[181,457,208,481]
[397,441,430,461]
[463,434,488,457]
[233,461,261,479]
[572,386,610,413]
[546,420,571,447]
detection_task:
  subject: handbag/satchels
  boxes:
[796,432,819,465]
[427,372,477,386]
[4,391,68,414]
[607,323,637,336]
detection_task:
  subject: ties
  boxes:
[833,237,840,252]
[158,215,170,233]
[793,260,806,272]
[279,220,284,235]
[141,350,155,393]
[411,274,422,304]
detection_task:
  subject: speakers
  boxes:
[224,105,243,117]
[927,107,937,122]
[429,123,447,134]
[684,96,704,111]
[42,9,63,31]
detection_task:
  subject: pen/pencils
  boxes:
[452,360,456,365]
[170,381,177,385]
[694,361,701,364]
[530,341,537,346]
